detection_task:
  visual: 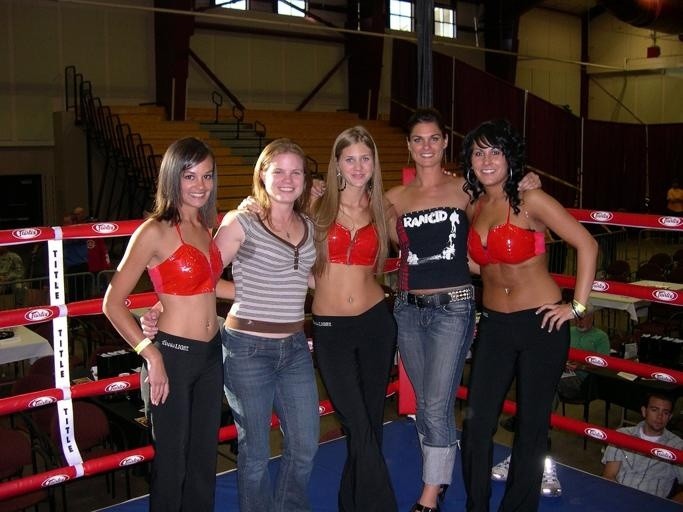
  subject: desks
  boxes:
[0,326,51,371]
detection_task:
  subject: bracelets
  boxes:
[569,295,587,319]
[132,336,152,355]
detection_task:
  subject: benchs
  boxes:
[95,96,464,222]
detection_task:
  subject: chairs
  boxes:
[0,350,133,510]
[548,251,682,452]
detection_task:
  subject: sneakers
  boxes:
[540,456,561,498]
[492,455,511,481]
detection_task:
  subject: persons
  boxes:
[236,126,399,512]
[465,123,599,512]
[139,136,314,512]
[665,176,683,216]
[102,137,235,512]
[601,390,683,497]
[0,206,111,303]
[500,302,611,449]
[309,109,543,512]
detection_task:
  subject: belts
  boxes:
[407,289,472,307]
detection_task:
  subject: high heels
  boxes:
[412,485,447,512]
